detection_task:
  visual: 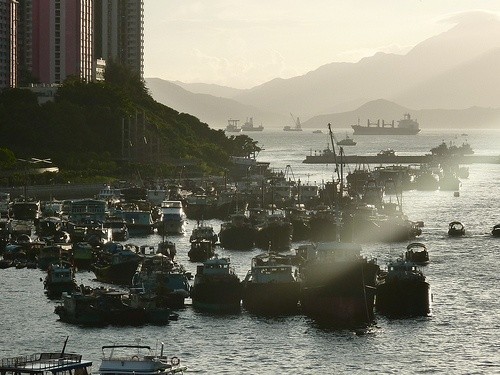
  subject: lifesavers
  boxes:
[171,356,180,366]
[111,254,122,265]
[133,294,138,301]
[131,355,139,361]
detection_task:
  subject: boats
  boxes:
[336,135,357,146]
[0,122,476,336]
[224,119,243,132]
[491,224,500,237]
[312,129,323,134]
[240,116,265,132]
[90,339,188,375]
[350,113,422,136]
[0,336,93,375]
[447,221,466,236]
[283,113,304,131]
